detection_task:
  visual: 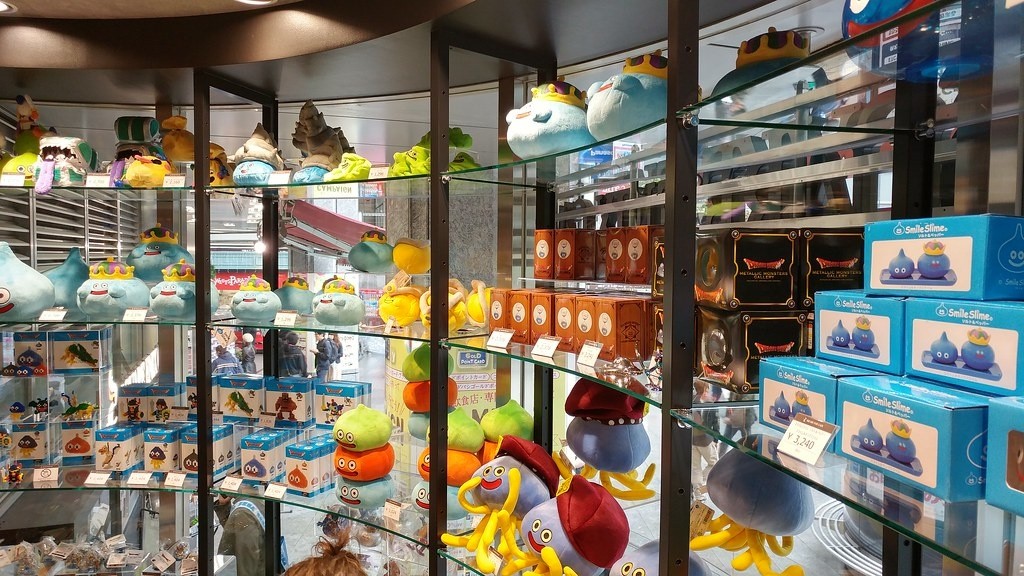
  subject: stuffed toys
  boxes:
[688,433,815,576]
[417,278,493,336]
[0,223,431,323]
[552,378,657,499]
[401,342,458,440]
[478,440,497,464]
[331,404,395,508]
[418,448,481,487]
[479,399,534,442]
[426,406,485,453]
[411,481,473,521]
[0,96,328,186]
[440,434,630,576]
[502,26,809,158]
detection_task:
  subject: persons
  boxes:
[211,345,244,374]
[214,494,265,576]
[691,378,757,500]
[309,331,332,382]
[238,333,257,374]
[278,330,313,378]
[327,333,343,381]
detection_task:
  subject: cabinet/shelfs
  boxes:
[0,0,1024,576]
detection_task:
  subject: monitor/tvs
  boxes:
[876,170,893,209]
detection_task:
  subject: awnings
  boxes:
[282,201,388,260]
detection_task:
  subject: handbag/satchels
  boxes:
[280,536,288,573]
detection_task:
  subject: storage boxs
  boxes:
[489,224,665,363]
[760,213,1024,518]
[652,228,863,394]
[95,372,372,498]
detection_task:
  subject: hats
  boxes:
[243,333,254,342]
